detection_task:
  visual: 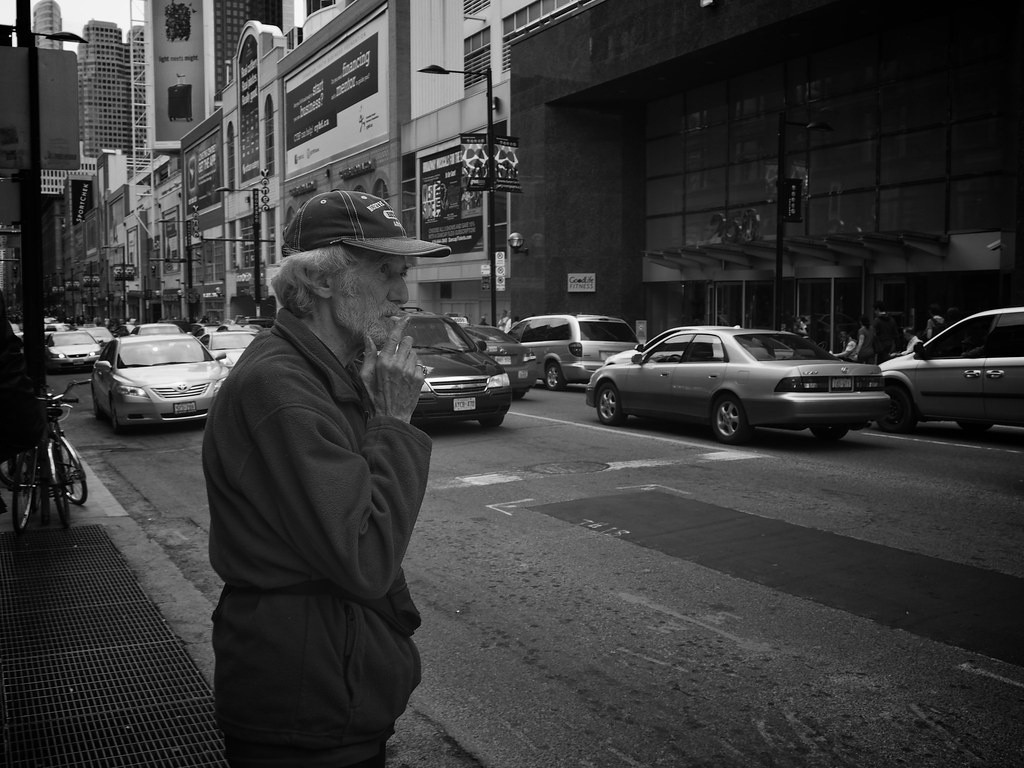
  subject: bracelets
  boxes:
[855,352,858,355]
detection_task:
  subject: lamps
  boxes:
[507,232,529,255]
[216,286,225,297]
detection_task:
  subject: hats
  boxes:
[281,188,451,258]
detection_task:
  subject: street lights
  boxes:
[417,65,497,328]
[215,186,262,317]
[74,260,93,323]
[158,219,194,324]
[775,111,836,330]
[101,246,126,324]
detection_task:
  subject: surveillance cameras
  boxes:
[988,239,1003,249]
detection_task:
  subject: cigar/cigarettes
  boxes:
[390,316,401,322]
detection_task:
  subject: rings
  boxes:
[415,364,427,375]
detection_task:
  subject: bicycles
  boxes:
[46,378,93,530]
[0,394,80,536]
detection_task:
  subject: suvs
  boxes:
[355,306,513,429]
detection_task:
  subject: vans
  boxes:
[506,314,641,392]
[876,307,1024,435]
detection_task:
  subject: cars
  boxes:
[199,332,258,368]
[45,331,104,374]
[110,325,136,338]
[158,320,241,339]
[585,327,890,446]
[70,324,114,347]
[604,325,794,366]
[236,316,274,329]
[90,333,229,436]
[11,317,70,338]
[806,313,859,352]
[446,324,539,401]
[129,324,193,336]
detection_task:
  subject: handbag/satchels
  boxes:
[872,331,881,353]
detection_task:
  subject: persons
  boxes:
[78,314,110,327]
[497,309,509,330]
[0,291,46,514]
[961,328,991,357]
[927,304,948,357]
[202,190,452,768]
[891,327,923,357]
[853,306,898,365]
[829,331,857,363]
[478,316,489,326]
